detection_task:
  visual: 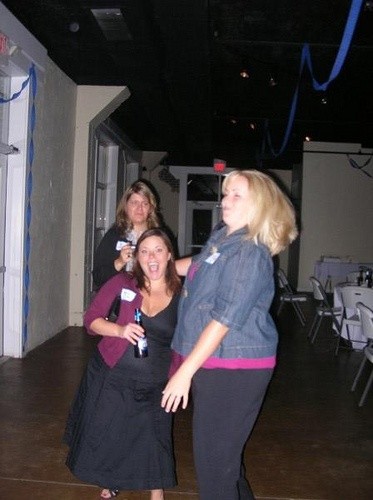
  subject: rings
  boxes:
[128,249,130,254]
[128,254,130,257]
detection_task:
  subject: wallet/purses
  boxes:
[107,293,121,322]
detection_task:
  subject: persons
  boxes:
[160,169,298,500]
[91,181,160,345]
[65,225,185,500]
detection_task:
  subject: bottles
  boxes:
[133,308,149,358]
[125,244,134,272]
[357,269,373,288]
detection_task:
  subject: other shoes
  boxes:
[100,488,118,499]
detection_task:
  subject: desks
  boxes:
[311,259,373,301]
[331,286,367,348]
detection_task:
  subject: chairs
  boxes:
[275,268,309,327]
[336,282,373,356]
[308,275,350,349]
[350,302,373,407]
